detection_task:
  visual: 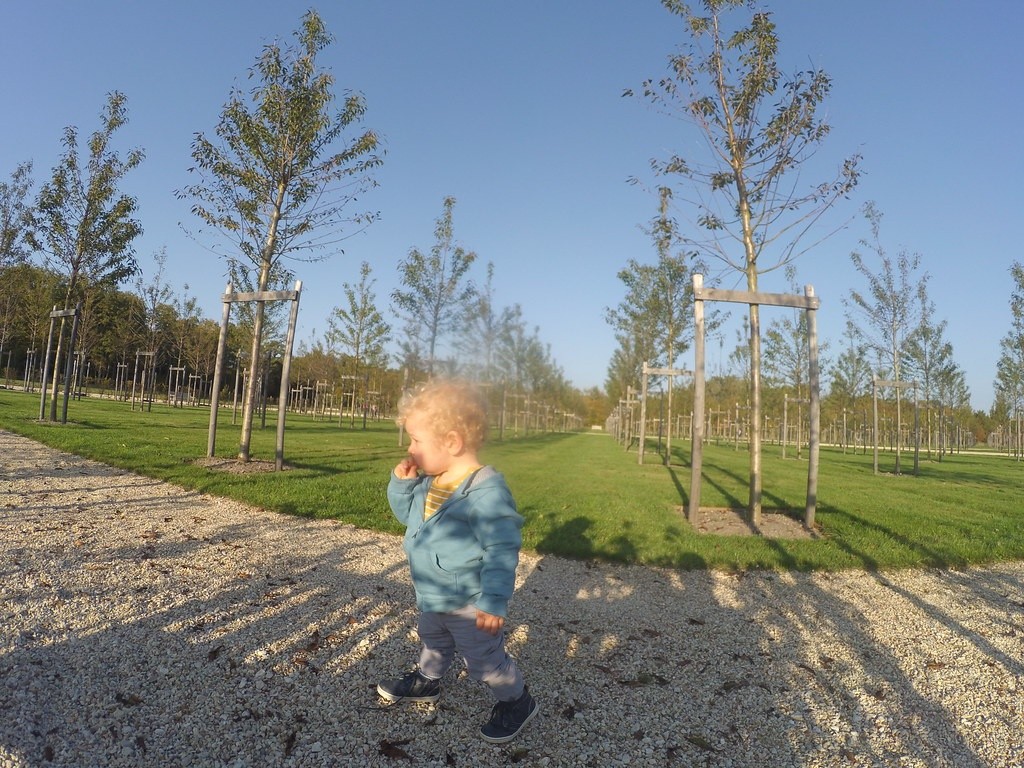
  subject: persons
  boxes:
[377,375,540,743]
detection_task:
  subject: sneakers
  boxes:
[377,668,441,702]
[479,684,539,743]
